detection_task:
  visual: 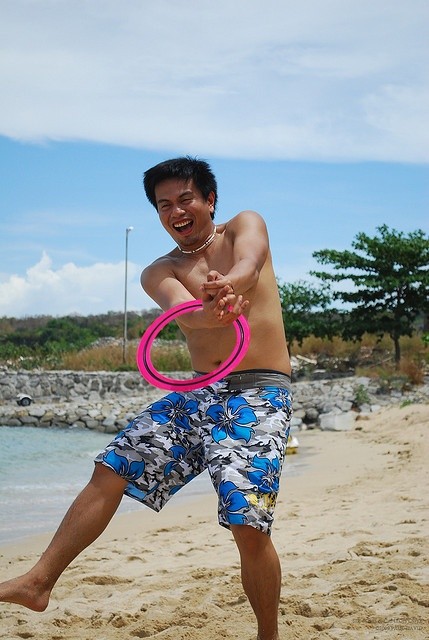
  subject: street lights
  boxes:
[123,227,134,365]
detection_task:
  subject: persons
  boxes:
[0,156,292,640]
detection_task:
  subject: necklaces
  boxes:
[176,225,217,253]
